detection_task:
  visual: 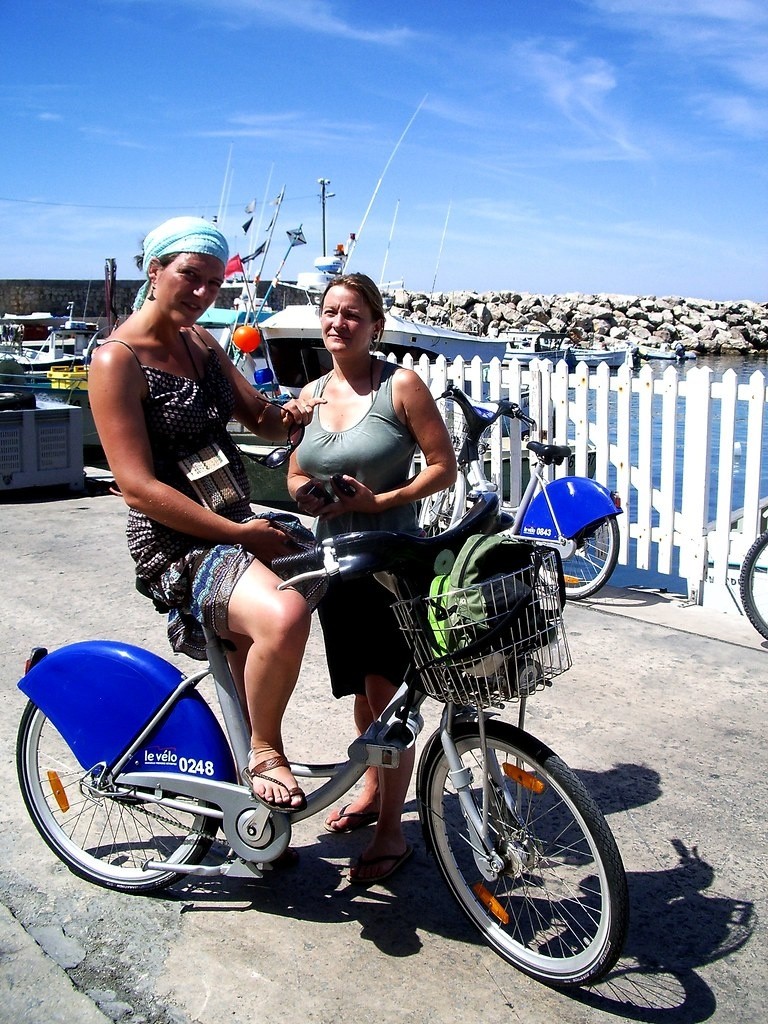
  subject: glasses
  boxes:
[228,396,304,468]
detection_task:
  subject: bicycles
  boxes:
[14,481,630,991]
[413,378,624,601]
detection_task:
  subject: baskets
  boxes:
[389,551,573,709]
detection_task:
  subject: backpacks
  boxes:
[422,530,567,693]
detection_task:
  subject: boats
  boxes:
[1,86,696,503]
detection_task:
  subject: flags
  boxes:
[223,255,243,279]
[242,217,252,235]
[245,201,254,214]
[265,217,274,232]
[269,194,281,208]
[241,241,266,264]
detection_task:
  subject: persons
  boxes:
[285,275,456,883]
[89,214,327,873]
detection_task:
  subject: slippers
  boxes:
[241,755,307,813]
[346,843,412,884]
[270,846,300,870]
[324,801,380,832]
